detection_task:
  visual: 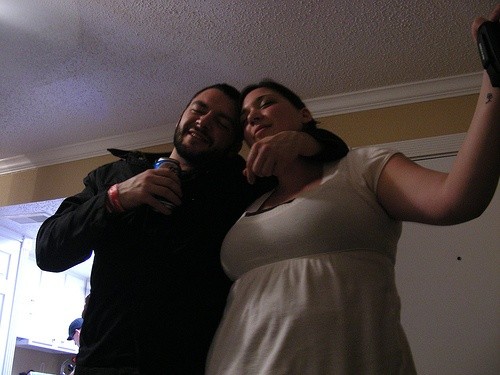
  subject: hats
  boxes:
[66,317,84,341]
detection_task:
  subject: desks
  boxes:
[11,341,79,375]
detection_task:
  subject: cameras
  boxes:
[476,13,500,90]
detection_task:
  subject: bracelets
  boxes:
[106,184,127,215]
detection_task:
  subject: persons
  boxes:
[30,82,352,375]
[202,2,500,375]
[60,317,85,375]
